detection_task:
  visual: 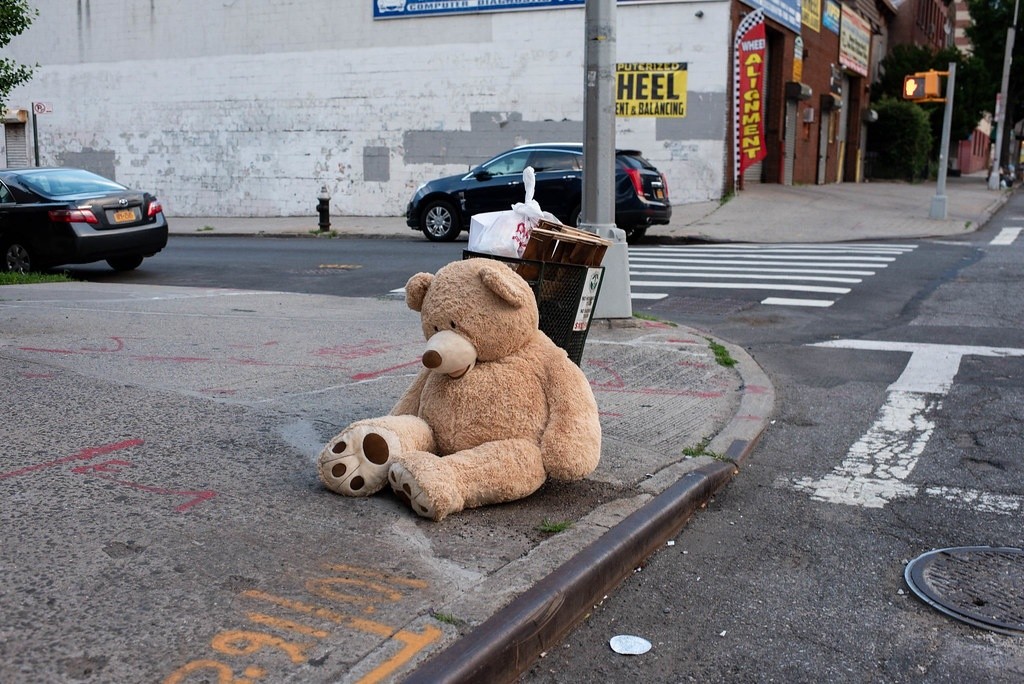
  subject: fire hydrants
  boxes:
[316,184,331,231]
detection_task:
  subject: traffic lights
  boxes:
[902,72,940,98]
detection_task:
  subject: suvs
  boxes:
[406,142,672,244]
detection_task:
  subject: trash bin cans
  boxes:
[463,250,607,368]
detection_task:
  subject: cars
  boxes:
[0,165,168,277]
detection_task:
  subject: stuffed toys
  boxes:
[317,258,602,524]
